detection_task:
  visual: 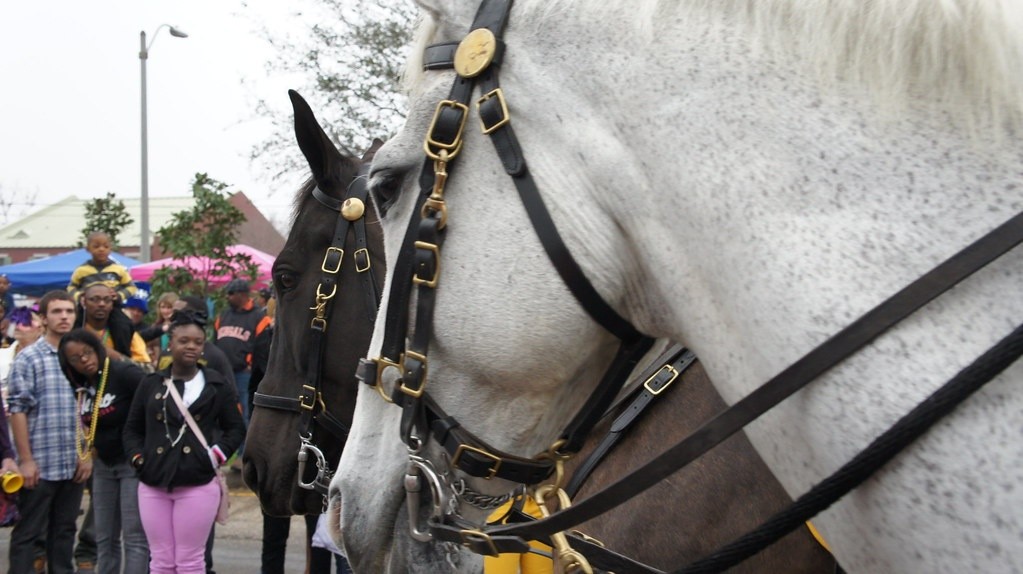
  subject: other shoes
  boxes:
[32,560,45,574]
[77,562,95,574]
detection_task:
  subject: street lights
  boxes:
[139,24,188,263]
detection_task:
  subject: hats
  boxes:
[225,279,247,292]
[120,282,151,314]
[257,288,271,300]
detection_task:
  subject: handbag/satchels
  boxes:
[215,473,230,525]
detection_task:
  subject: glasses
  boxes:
[85,295,111,303]
[67,346,92,366]
[228,291,235,295]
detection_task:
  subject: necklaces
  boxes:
[75,358,109,463]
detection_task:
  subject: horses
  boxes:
[242,0,1023,574]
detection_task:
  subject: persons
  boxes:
[0,277,49,574]
[157,298,239,574]
[57,329,152,574]
[67,233,179,357]
[213,280,272,470]
[121,322,248,574]
[8,290,93,574]
[33,284,149,574]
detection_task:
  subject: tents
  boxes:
[1,248,149,294]
[130,245,277,291]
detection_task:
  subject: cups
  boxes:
[0,469,24,494]
